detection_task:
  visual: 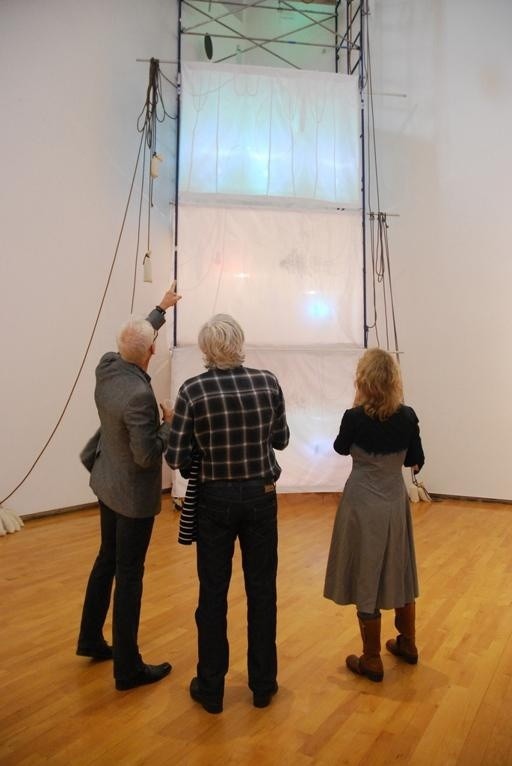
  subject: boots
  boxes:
[386,600,417,665]
[345,611,384,681]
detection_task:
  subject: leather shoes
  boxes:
[254,680,278,707]
[190,677,223,714]
[115,662,171,690]
[76,644,113,660]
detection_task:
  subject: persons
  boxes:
[164,313,292,713]
[322,346,425,682]
[74,280,183,690]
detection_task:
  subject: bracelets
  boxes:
[155,306,166,315]
